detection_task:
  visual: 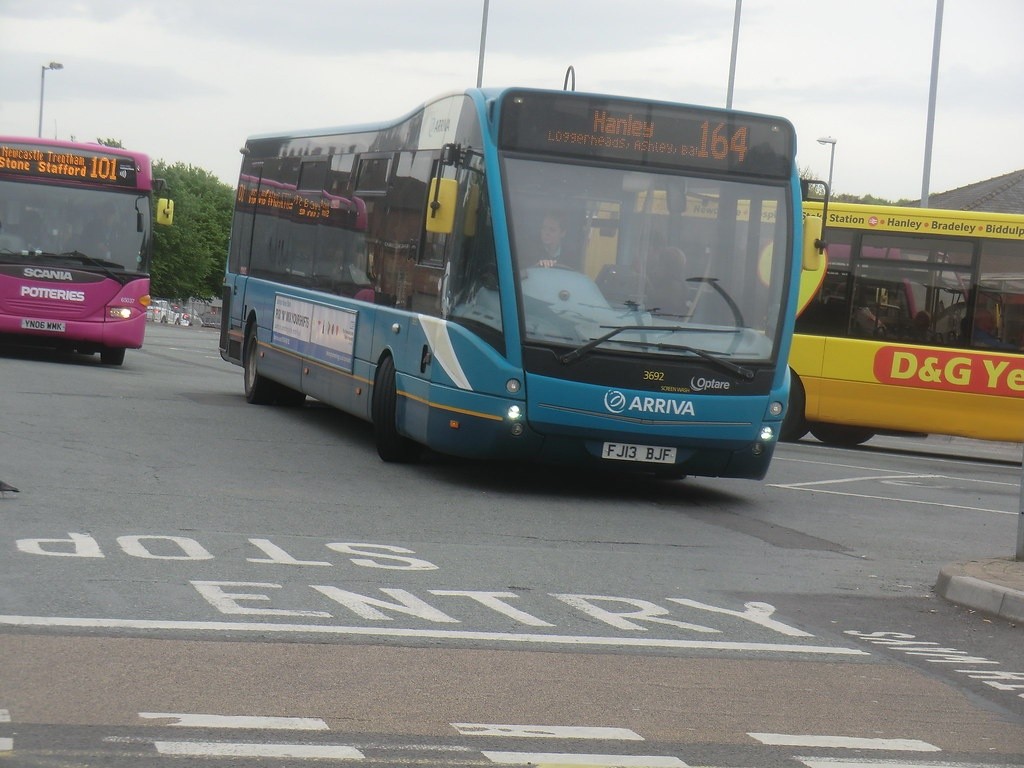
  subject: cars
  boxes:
[145,299,220,327]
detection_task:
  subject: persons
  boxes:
[895,311,930,344]
[7,209,51,253]
[846,288,888,340]
[644,246,687,310]
[971,310,1021,351]
[519,208,579,272]
[65,220,109,259]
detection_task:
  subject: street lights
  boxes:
[39,61,65,141]
[816,138,838,201]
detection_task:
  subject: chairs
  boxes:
[872,324,1003,348]
[597,262,646,311]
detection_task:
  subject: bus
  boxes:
[0,136,174,365]
[585,189,1024,446]
[220,88,829,482]
